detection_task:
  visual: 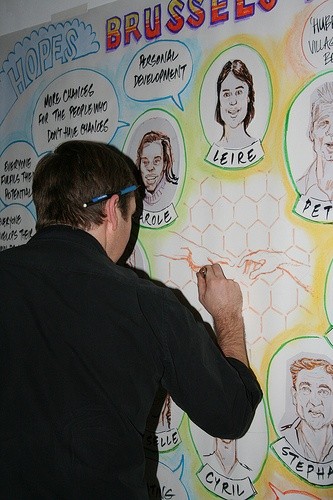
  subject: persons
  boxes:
[0,140,263,500]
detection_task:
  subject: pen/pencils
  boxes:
[199,267,207,281]
[84,184,141,209]
